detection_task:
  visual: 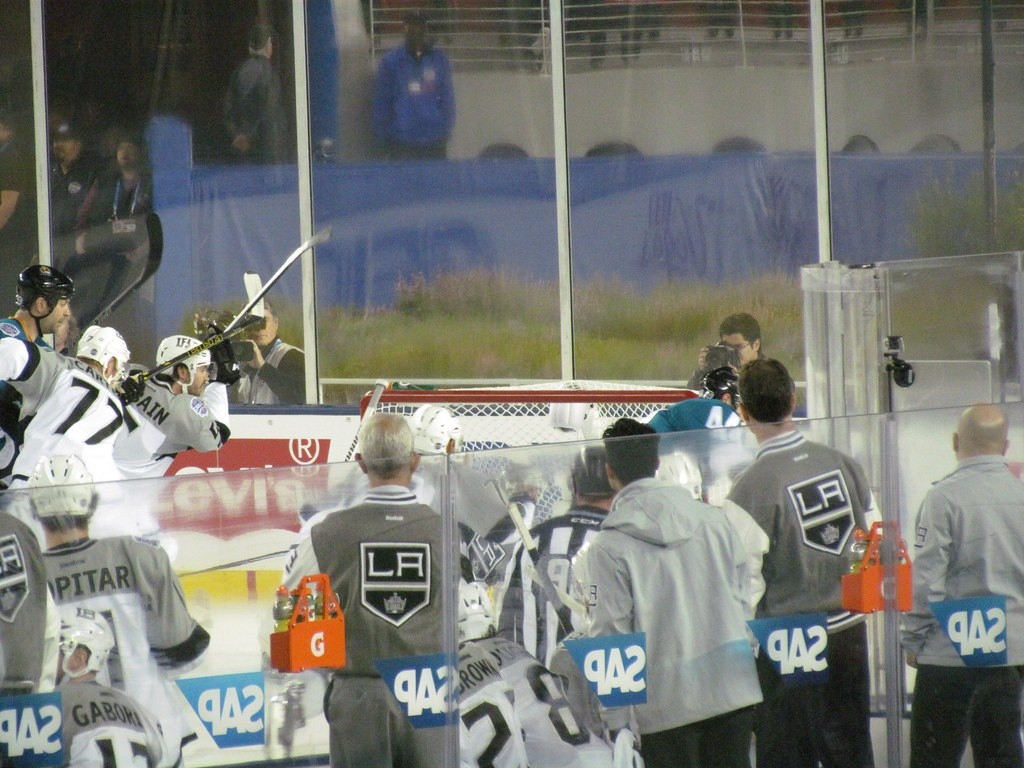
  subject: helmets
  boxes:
[60,610,114,677]
[457,580,498,646]
[155,334,210,385]
[701,367,742,412]
[77,325,130,369]
[409,404,462,464]
[16,264,74,309]
[28,454,95,518]
[569,446,615,497]
[654,452,703,503]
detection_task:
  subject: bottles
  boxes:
[273,585,337,633]
[849,529,907,574]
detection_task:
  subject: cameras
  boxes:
[706,341,739,370]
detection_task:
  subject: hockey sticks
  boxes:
[131,269,266,383]
[176,497,320,578]
[324,378,392,511]
[57,212,164,356]
[484,477,575,634]
[222,222,335,334]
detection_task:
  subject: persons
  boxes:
[0,109,152,348]
[901,403,1024,768]
[283,404,539,768]
[570,418,764,768]
[0,512,62,694]
[29,451,212,768]
[685,313,765,391]
[53,606,164,768]
[495,444,617,672]
[222,25,285,167]
[455,577,611,768]
[648,367,760,507]
[721,358,884,768]
[0,265,232,546]
[369,14,456,160]
[223,299,306,405]
